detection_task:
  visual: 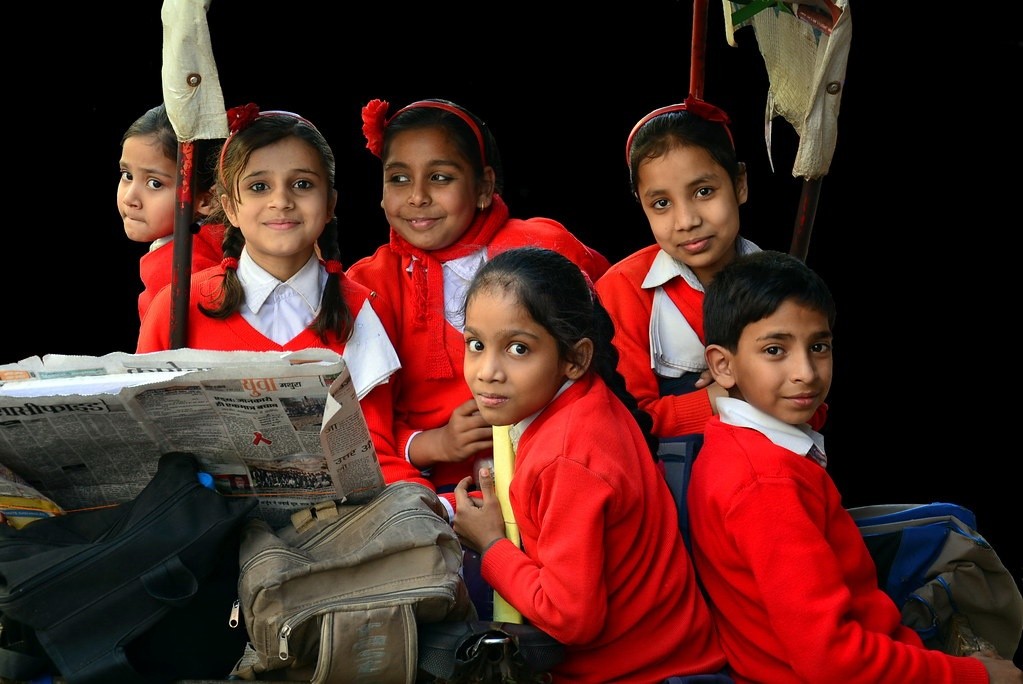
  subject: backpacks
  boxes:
[227,479,477,684]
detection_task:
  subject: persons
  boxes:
[133,111,436,492]
[439,244,726,683]
[593,103,828,439]
[687,252,1022,684]
[344,99,612,493]
[115,104,227,323]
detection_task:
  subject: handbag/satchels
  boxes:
[0,448,251,684]
[418,614,565,684]
[845,501,1023,663]
